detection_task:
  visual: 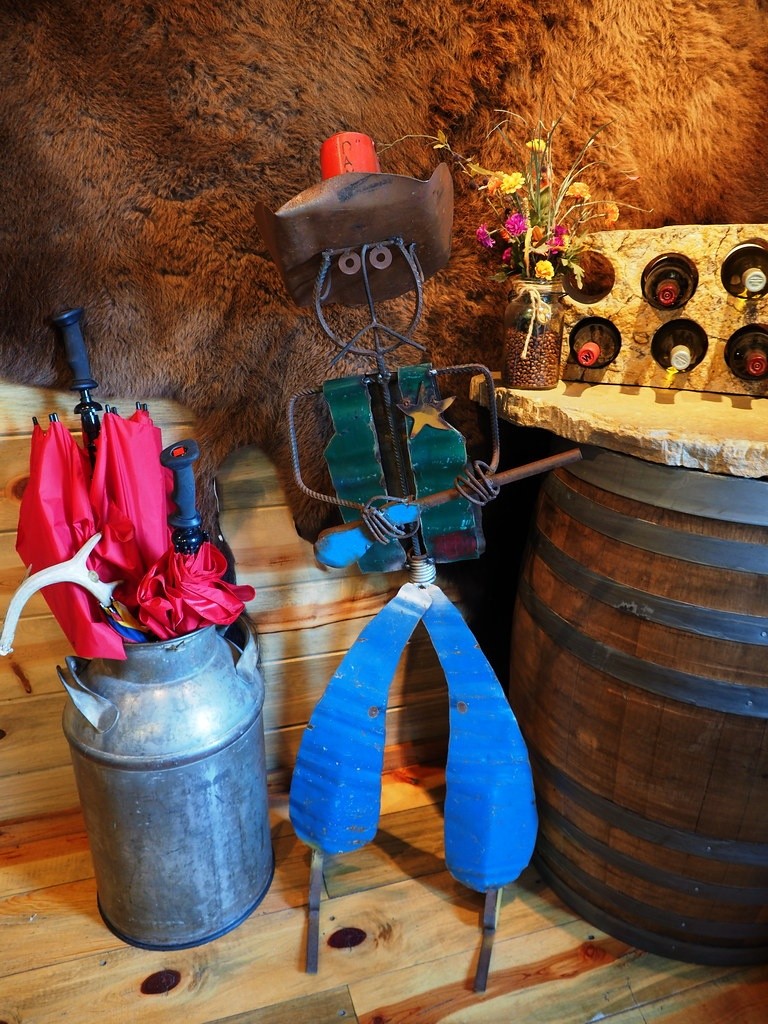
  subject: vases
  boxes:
[500,282,570,390]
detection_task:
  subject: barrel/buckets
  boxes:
[57,617,277,950]
[508,447,768,966]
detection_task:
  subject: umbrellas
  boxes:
[1,307,256,661]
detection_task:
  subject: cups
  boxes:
[320,131,381,181]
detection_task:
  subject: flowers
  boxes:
[373,86,657,282]
